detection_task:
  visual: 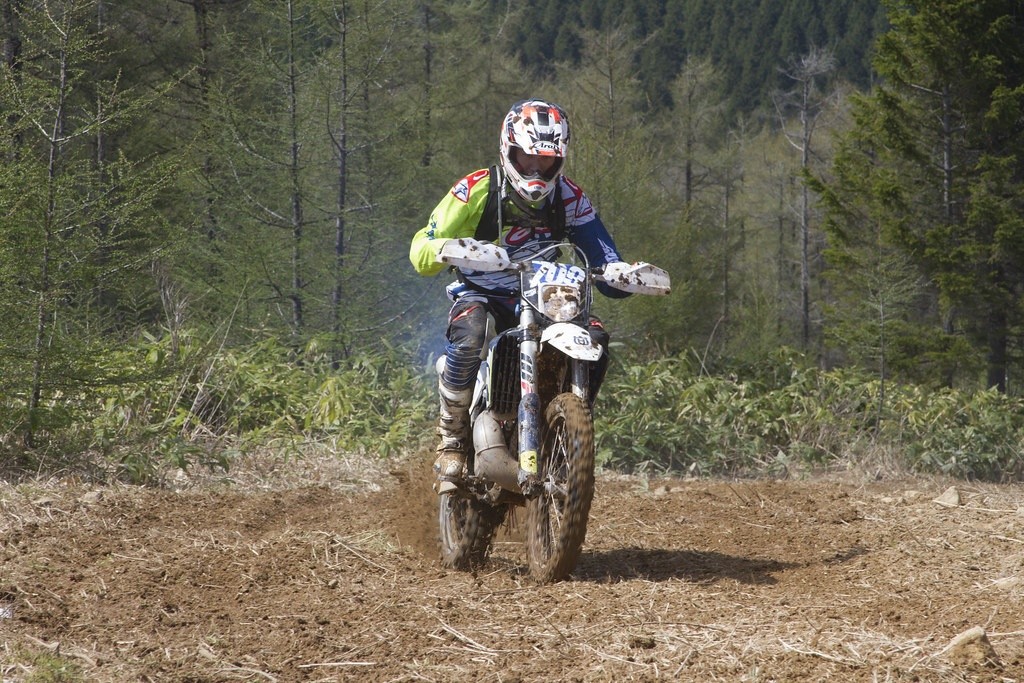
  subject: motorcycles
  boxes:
[434,237,671,587]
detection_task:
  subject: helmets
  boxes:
[499,98,570,202]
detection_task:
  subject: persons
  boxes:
[410,99,647,483]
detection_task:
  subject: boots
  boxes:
[432,370,473,481]
